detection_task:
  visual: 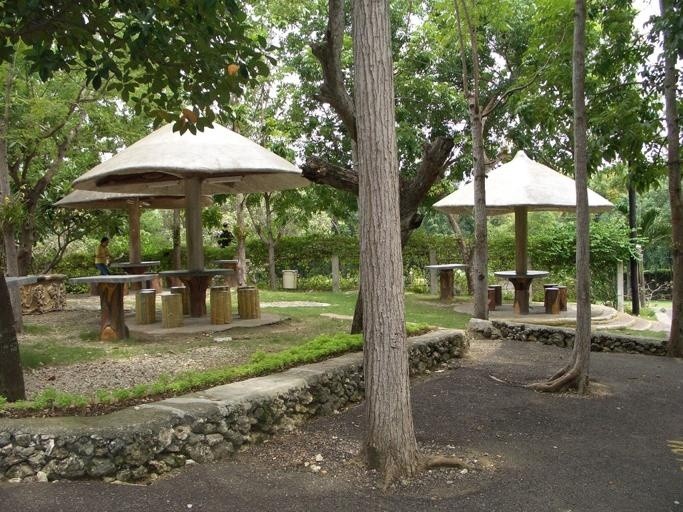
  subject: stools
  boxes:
[487,284,501,310]
[236,285,261,320]
[543,283,568,315]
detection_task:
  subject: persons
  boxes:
[218,222,235,248]
[95,237,113,275]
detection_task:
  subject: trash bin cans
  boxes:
[282,270,298,290]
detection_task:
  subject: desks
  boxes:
[424,264,467,304]
[494,270,549,314]
[69,259,250,342]
